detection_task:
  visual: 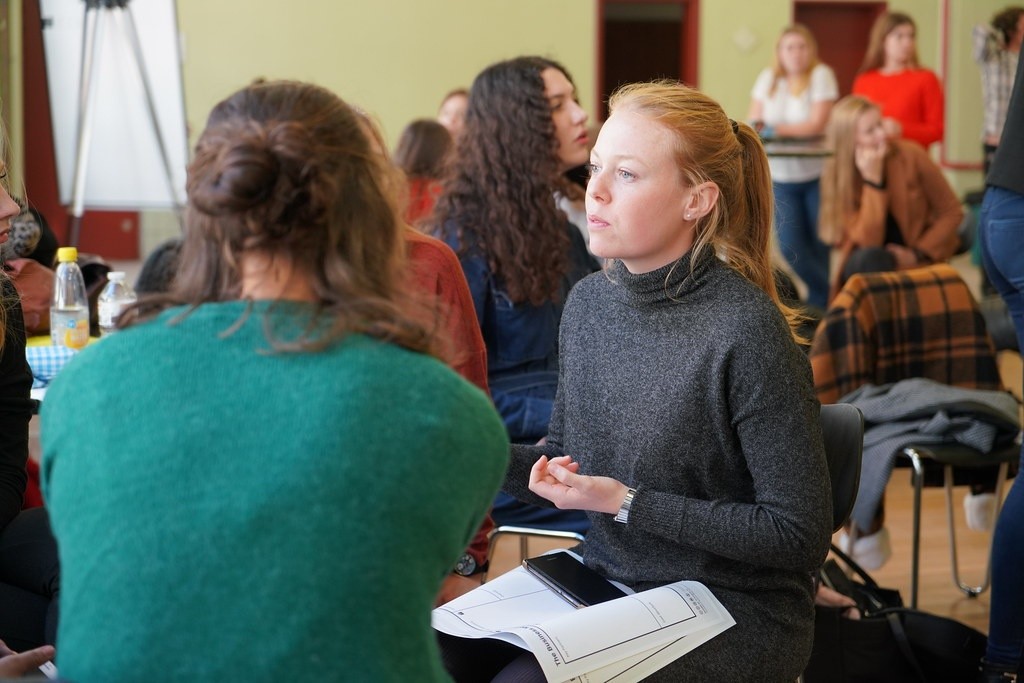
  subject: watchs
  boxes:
[455,553,489,576]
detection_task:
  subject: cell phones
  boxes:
[521,551,628,609]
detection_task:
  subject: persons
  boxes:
[971,7,1024,171]
[436,83,832,683]
[817,93,966,305]
[977,39,1024,360]
[0,119,60,661]
[747,22,839,311]
[852,12,945,151]
[419,55,605,536]
[40,79,511,683]
[396,89,470,226]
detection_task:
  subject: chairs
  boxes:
[481,403,1023,683]
[808,263,1024,608]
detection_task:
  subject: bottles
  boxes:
[50,247,89,351]
[98,272,139,337]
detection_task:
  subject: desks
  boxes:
[757,139,835,156]
[28,331,100,413]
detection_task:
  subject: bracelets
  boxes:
[614,489,637,523]
